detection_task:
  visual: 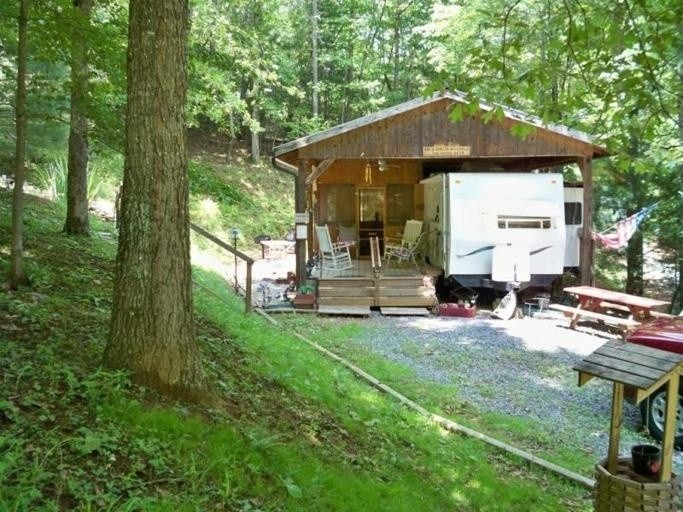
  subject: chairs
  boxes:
[313,217,437,274]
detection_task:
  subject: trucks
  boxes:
[412,171,583,304]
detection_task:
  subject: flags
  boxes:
[590,205,660,250]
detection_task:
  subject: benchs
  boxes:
[597,301,675,323]
[547,303,641,344]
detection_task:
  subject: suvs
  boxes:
[623,316,683,448]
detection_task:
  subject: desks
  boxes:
[548,286,676,342]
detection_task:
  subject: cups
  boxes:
[630,444,660,478]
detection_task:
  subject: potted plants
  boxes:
[631,425,661,476]
